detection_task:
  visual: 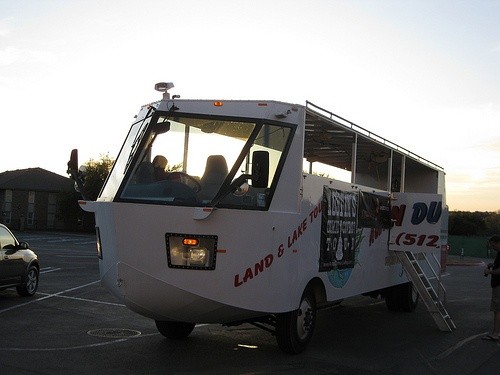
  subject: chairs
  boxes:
[198,155,228,200]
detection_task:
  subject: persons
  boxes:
[481,235,500,341]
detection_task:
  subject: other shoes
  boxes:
[482,333,500,340]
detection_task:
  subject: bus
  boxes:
[78,83,455,351]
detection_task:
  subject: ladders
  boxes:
[394,251,457,331]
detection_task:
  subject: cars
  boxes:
[0,224,40,298]
